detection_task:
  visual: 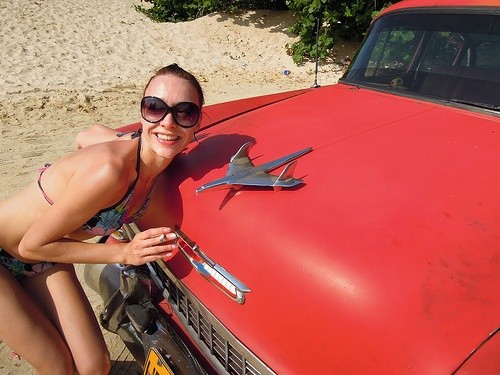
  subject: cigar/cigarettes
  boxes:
[160,234,164,241]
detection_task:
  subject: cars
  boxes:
[83,0,499,375]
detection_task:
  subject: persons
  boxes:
[0,63,205,375]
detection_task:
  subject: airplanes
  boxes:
[194,141,313,196]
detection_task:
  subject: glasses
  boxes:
[140,96,201,128]
